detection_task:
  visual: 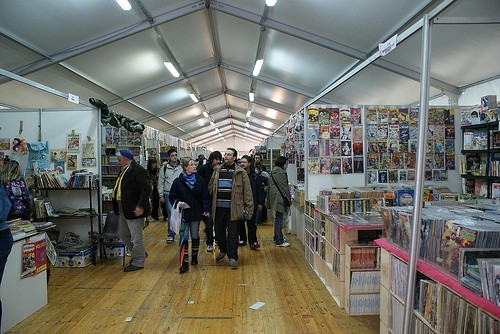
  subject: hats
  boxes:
[119,148,133,160]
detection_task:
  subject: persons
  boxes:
[268,156,293,247]
[147,148,268,252]
[112,149,152,272]
[168,158,210,273]
[208,148,254,269]
[0,178,13,334]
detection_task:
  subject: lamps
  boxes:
[115,0,278,143]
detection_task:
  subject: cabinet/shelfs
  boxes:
[33,174,102,266]
[304,198,500,334]
[101,144,143,214]
[460,120,500,199]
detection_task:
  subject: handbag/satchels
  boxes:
[283,197,291,207]
[170,200,182,235]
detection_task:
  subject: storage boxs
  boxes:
[103,241,126,259]
[52,245,93,268]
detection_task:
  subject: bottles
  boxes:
[242,209,249,220]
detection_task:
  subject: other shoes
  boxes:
[283,236,287,242]
[251,242,260,250]
[212,240,218,246]
[238,240,246,246]
[276,242,290,247]
[123,265,144,272]
[228,259,238,269]
[207,245,213,252]
[165,218,169,221]
[216,252,226,262]
[166,237,174,244]
[126,252,149,265]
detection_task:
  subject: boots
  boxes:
[178,241,190,274]
[191,238,200,265]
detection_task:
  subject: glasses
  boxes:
[285,162,289,166]
[170,154,178,157]
[117,158,125,161]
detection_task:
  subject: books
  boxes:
[459,107,500,198]
[102,155,141,176]
[5,218,56,235]
[308,106,455,183]
[304,186,455,316]
[33,169,98,188]
[34,197,95,217]
[379,205,500,334]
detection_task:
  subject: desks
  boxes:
[0,225,56,334]
